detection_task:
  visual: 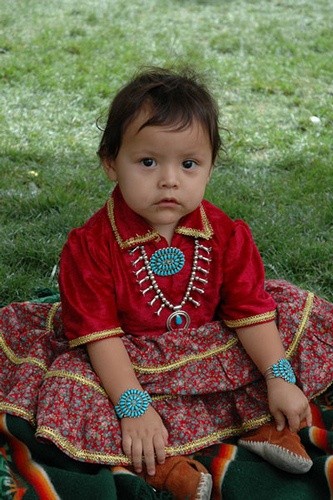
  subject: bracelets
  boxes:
[114,387,153,419]
[264,359,297,384]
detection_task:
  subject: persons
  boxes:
[0,66,333,500]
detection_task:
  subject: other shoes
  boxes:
[144,457,212,500]
[237,420,313,474]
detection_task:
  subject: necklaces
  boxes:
[129,236,213,331]
